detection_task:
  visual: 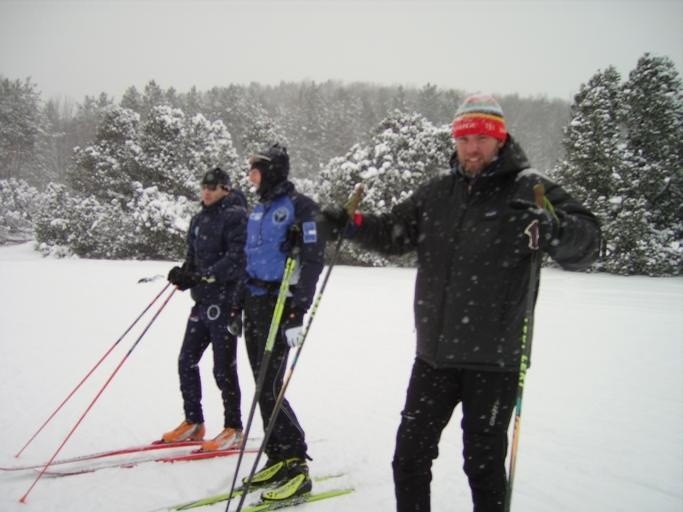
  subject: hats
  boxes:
[249,145,290,195]
[200,168,232,187]
[449,91,507,142]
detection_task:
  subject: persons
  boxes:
[161,167,248,450]
[226,144,325,501]
[324,94,601,512]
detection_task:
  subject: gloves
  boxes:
[225,307,241,337]
[283,314,306,348]
[503,200,555,257]
[167,265,202,292]
[315,204,346,241]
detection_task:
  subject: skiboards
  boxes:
[150,473,355,511]
[1,441,260,475]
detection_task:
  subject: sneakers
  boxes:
[201,427,244,451]
[241,456,287,488]
[163,420,205,442]
[259,463,313,502]
